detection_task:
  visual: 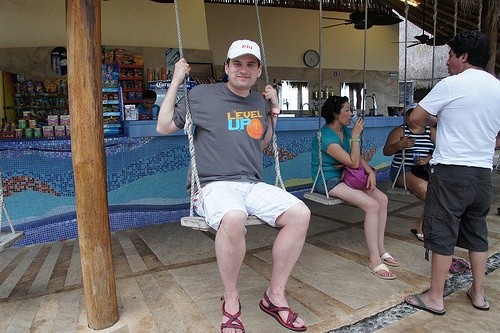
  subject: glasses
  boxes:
[333,95,337,102]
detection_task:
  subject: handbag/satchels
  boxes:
[341,162,378,191]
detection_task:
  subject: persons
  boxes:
[311,96,399,280]
[403,28,500,316]
[384,106,437,242]
[137,90,161,121]
[157,41,311,332]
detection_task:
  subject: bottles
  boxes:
[312,85,334,99]
[167,70,173,80]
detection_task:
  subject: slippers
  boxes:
[382,251,399,267]
[466,288,490,310]
[404,293,446,315]
[410,227,424,241]
[367,263,397,279]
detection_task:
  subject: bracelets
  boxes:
[367,170,372,174]
[350,138,361,142]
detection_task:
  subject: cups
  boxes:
[412,152,420,164]
[351,114,365,127]
[151,108,158,120]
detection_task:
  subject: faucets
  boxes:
[365,92,377,117]
[301,103,312,116]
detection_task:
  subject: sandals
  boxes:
[219,297,245,333]
[259,292,307,330]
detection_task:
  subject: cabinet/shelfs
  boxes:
[102,86,125,137]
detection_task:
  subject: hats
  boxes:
[403,103,417,112]
[226,39,262,63]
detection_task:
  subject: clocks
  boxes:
[304,50,320,67]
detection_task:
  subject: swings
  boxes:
[453,0,482,36]
[386,0,438,196]
[175,1,286,232]
[304,0,367,205]
